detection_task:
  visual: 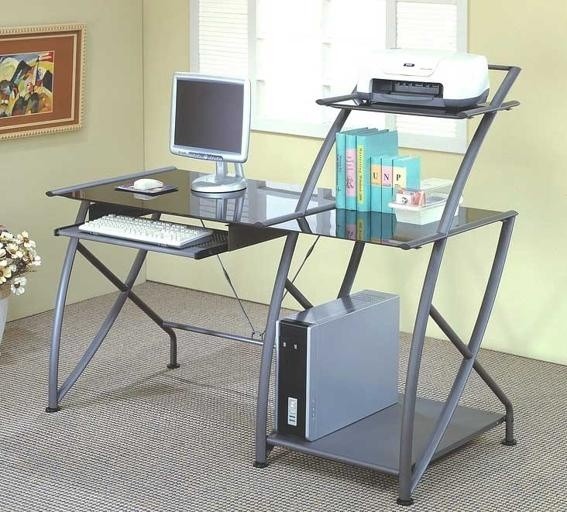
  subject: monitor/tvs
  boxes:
[168,71,252,194]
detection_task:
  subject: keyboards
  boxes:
[78,212,214,250]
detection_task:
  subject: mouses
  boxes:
[133,192,161,201]
[133,178,163,191]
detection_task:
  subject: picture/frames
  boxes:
[0,22,89,140]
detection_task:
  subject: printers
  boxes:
[354,47,491,109]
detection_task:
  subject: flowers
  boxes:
[0,223,44,296]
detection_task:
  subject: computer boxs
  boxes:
[274,287,402,443]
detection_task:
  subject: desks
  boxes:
[45,62,522,507]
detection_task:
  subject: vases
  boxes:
[0,288,10,344]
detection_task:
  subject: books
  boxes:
[335,209,397,241]
[334,126,422,214]
